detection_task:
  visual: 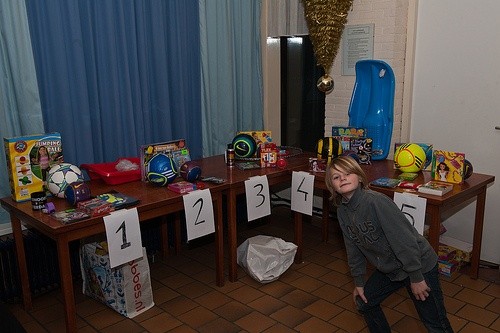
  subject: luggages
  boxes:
[0,227,62,305]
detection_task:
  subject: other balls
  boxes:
[46,162,84,198]
[393,143,426,173]
[145,152,178,185]
[232,133,258,159]
[316,136,343,164]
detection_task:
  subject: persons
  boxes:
[252,132,258,140]
[437,162,450,181]
[31,146,58,181]
[325,156,454,333]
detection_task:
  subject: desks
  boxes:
[189,149,312,282]
[0,179,229,333]
[307,160,496,284]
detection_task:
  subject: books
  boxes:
[418,180,453,196]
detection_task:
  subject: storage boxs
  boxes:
[140,139,186,181]
[84,156,141,186]
[316,127,373,165]
[236,130,277,166]
[4,131,64,202]
[437,242,466,277]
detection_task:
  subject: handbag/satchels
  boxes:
[79,238,155,320]
[237,235,298,284]
